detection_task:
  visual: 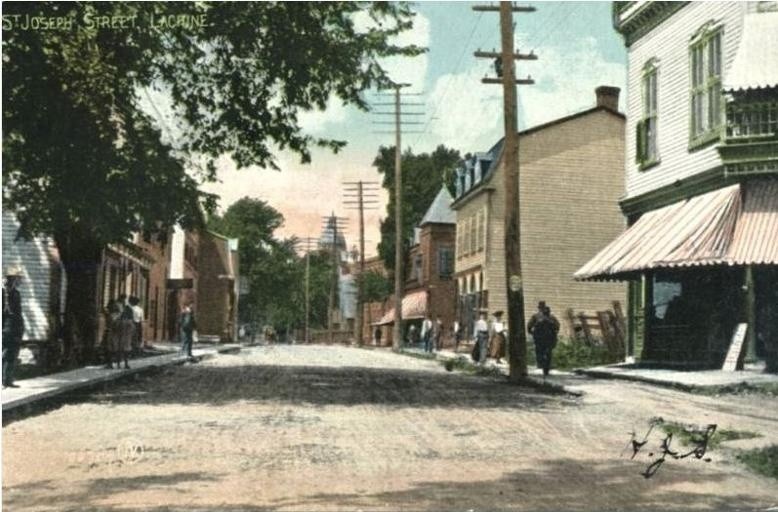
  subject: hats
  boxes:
[493,310,503,316]
[4,267,25,278]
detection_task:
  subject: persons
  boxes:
[407,303,559,374]
[178,300,196,357]
[101,294,145,368]
[2,267,23,388]
[375,326,381,347]
